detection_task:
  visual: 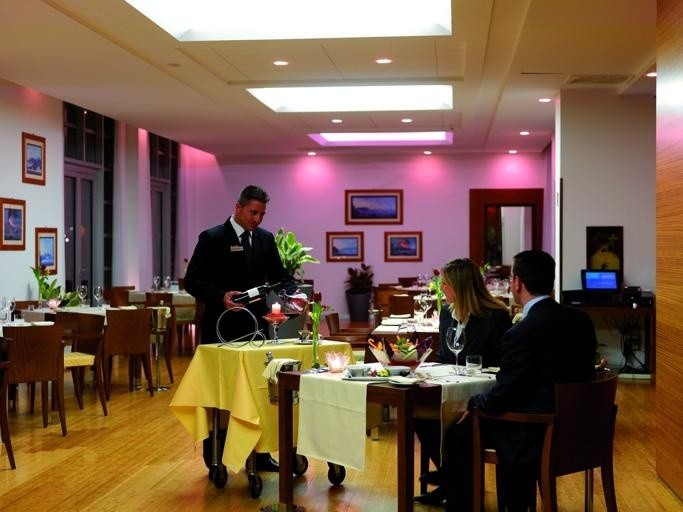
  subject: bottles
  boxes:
[278,289,309,313]
[232,282,281,305]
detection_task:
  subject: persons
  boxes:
[447,249,598,512]
[412,258,514,505]
[183,185,297,473]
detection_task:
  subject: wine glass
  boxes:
[0,295,16,326]
[490,278,511,297]
[445,327,465,376]
[77,285,87,308]
[413,295,437,328]
[92,286,103,310]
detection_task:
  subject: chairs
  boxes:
[322,280,520,362]
[471,371,618,512]
[0,278,199,469]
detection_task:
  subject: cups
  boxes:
[466,355,482,378]
[27,305,34,311]
[412,272,433,288]
[13,314,23,326]
[152,276,179,293]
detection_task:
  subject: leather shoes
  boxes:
[258,457,278,471]
[415,471,446,505]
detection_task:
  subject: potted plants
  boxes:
[341,262,371,322]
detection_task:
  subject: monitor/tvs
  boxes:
[581,269,621,295]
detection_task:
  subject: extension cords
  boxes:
[617,372,651,379]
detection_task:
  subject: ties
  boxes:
[239,230,251,254]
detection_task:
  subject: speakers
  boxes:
[625,285,642,306]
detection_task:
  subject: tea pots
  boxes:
[47,298,62,310]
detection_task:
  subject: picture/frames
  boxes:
[0,197,26,251]
[585,226,623,282]
[383,230,422,262]
[325,230,363,263]
[342,189,404,224]
[22,130,45,186]
[33,227,57,275]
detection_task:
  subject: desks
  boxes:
[277,362,499,512]
[198,338,354,498]
[565,287,654,376]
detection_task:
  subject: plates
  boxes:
[344,364,423,386]
